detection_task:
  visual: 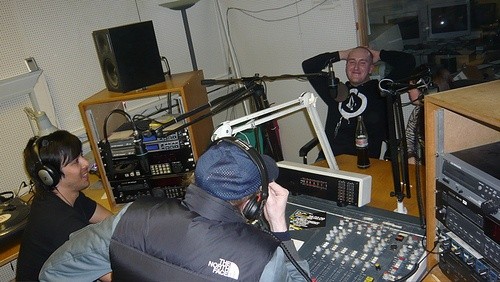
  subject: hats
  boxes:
[195,142,280,200]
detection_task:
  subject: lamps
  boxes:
[24,91,59,137]
[158,0,201,70]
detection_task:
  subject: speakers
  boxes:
[91,20,165,93]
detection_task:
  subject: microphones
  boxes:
[424,80,438,94]
[134,144,149,174]
[328,62,348,102]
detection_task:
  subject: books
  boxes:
[101,130,134,143]
[148,114,185,132]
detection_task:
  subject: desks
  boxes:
[0,151,124,268]
[309,153,428,219]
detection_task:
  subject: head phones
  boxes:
[205,136,269,223]
[32,136,58,187]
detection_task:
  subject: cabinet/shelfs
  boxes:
[78,69,215,213]
[424,78,500,282]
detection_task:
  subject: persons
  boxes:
[15,130,116,282]
[398,64,452,164]
[302,45,417,164]
[38,137,311,282]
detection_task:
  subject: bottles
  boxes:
[355,116,370,169]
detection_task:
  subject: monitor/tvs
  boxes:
[368,24,403,52]
[384,12,422,44]
[428,1,470,39]
